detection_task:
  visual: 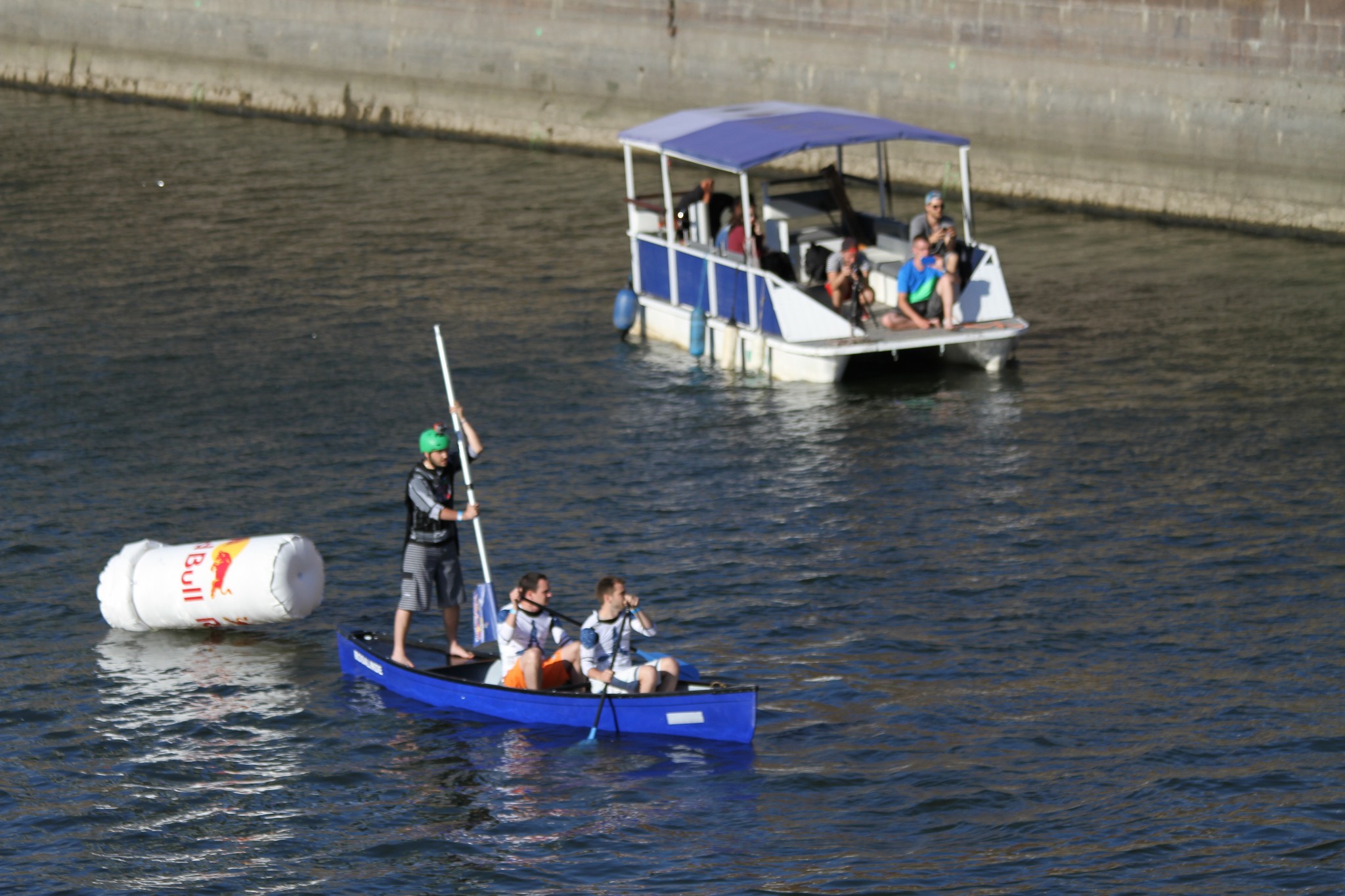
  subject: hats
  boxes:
[841,238,859,254]
[924,189,942,206]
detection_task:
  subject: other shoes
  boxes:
[860,314,869,322]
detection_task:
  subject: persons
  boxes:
[906,191,959,299]
[728,201,798,282]
[882,235,956,332]
[496,572,580,692]
[715,207,735,251]
[581,578,678,694]
[390,399,483,667]
[826,237,875,322]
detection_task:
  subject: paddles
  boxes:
[586,603,631,740]
[514,594,701,681]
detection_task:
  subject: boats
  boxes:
[599,104,1030,392]
[340,629,760,748]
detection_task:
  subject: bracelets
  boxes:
[630,608,640,614]
[461,420,466,424]
[509,608,517,615]
[456,510,462,522]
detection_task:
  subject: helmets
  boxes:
[419,429,450,454]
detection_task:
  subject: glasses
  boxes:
[932,203,944,209]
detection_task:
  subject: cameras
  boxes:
[850,263,862,277]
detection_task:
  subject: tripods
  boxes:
[845,281,878,339]
